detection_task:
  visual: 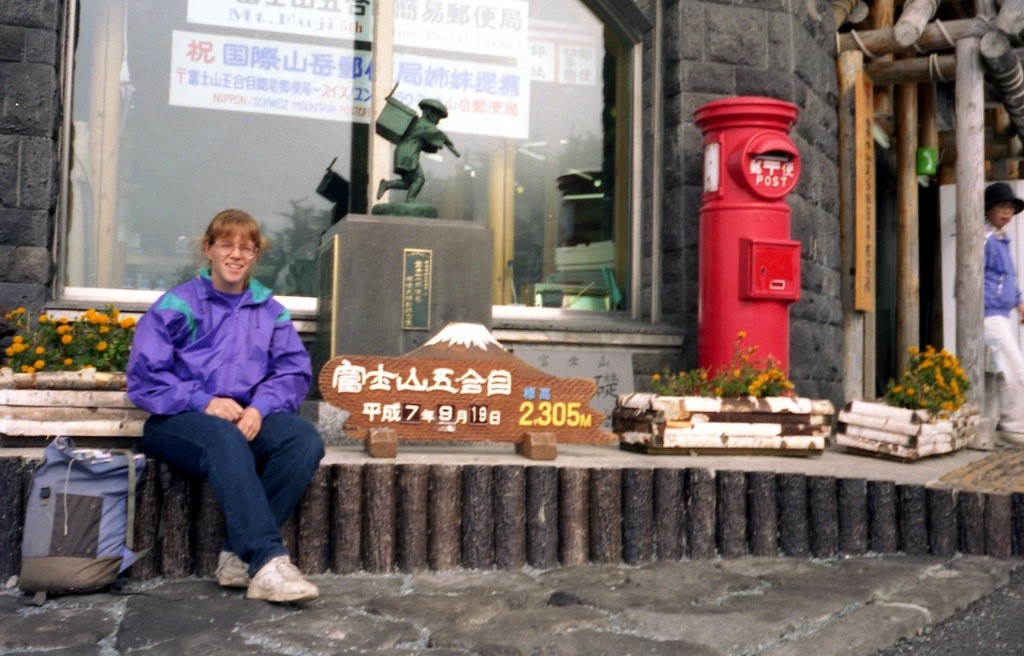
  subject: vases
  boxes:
[0,372,152,436]
[837,399,980,459]
[612,392,834,456]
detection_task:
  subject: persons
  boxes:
[984,182,1024,442]
[127,210,328,604]
[377,99,455,203]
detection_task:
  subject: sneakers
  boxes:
[246,555,320,602]
[213,550,251,592]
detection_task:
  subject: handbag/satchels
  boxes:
[19,436,146,607]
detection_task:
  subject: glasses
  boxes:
[216,240,258,259]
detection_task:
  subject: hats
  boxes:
[985,183,1024,217]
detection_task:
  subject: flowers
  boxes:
[886,344,973,425]
[1,306,131,373]
[651,330,794,396]
